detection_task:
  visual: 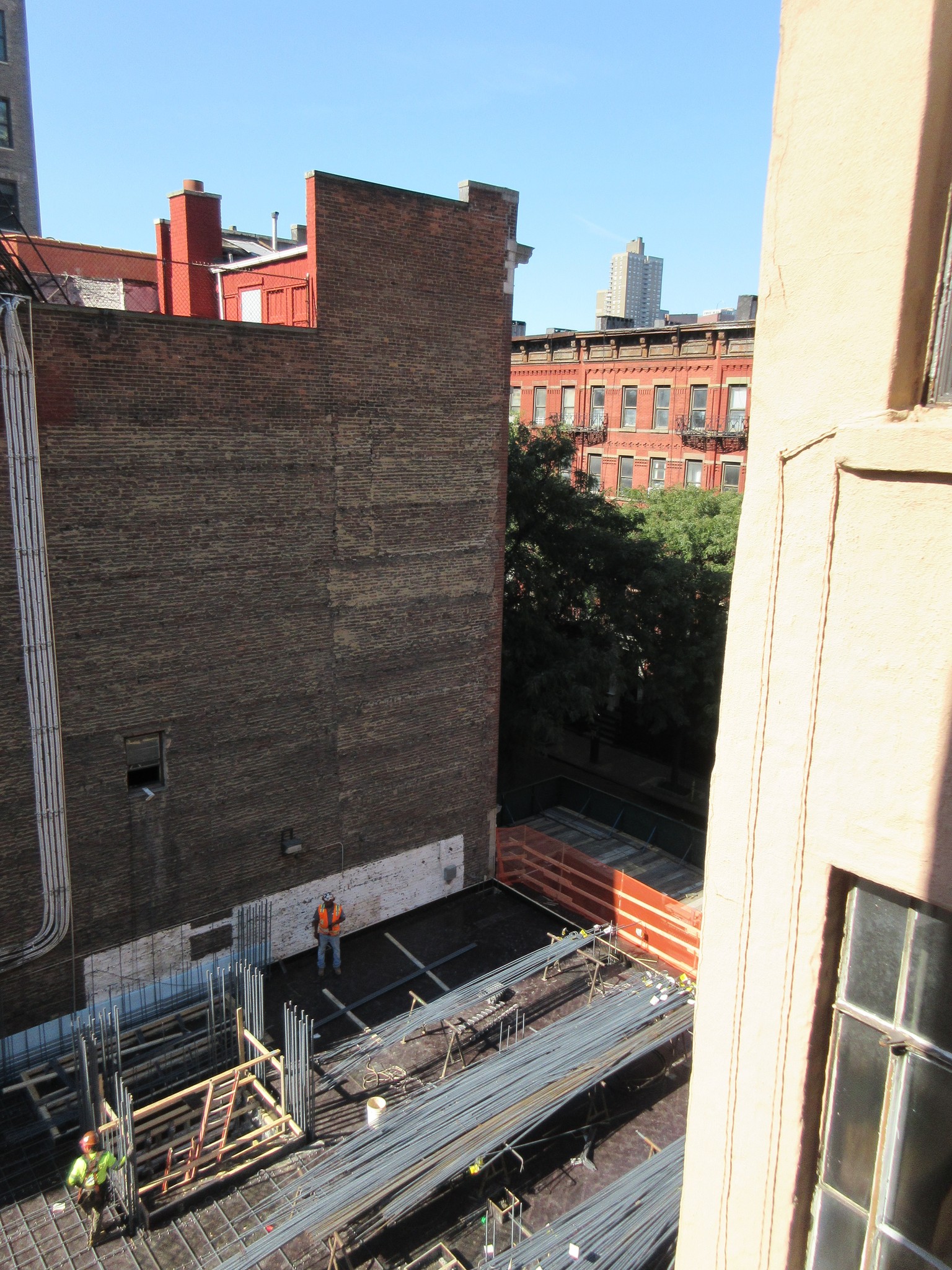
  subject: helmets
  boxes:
[322,892,335,902]
[79,1131,100,1147]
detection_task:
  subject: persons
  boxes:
[68,1131,134,1247]
[313,892,346,977]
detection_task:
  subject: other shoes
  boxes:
[87,1231,108,1246]
[317,968,325,976]
[335,967,341,976]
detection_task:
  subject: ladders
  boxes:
[191,1070,242,1181]
[162,1135,199,1191]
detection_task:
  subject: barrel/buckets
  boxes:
[367,1097,387,1126]
